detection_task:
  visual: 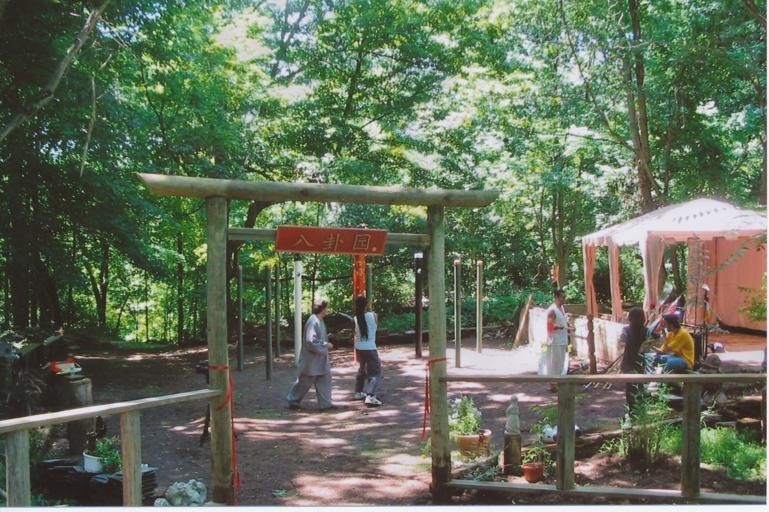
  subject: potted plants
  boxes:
[517,445,554,483]
[448,393,493,466]
[525,399,560,445]
[79,433,122,475]
[97,448,120,474]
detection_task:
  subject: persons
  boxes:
[284,298,341,412]
[644,311,696,390]
[351,295,384,406]
[544,289,577,392]
[504,394,522,436]
[615,306,654,421]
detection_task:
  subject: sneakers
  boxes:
[363,393,383,406]
[352,390,368,401]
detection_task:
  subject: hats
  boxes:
[661,312,681,321]
